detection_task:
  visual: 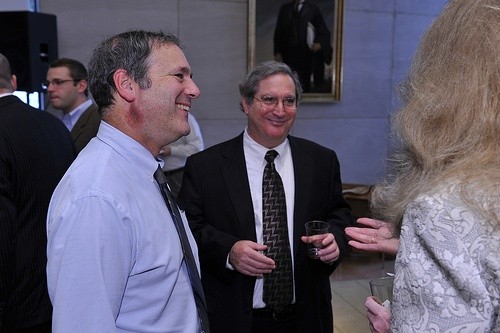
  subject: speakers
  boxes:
[0,11,59,93]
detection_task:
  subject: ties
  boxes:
[262,150,293,314]
[153,164,210,333]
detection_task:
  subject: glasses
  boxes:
[42,79,79,89]
[252,95,297,109]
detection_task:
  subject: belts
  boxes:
[253,305,296,318]
[163,168,185,176]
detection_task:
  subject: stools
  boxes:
[342,184,385,263]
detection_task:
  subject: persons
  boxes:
[157,113,205,194]
[0,53,79,333]
[46,57,101,155]
[345,0,500,333]
[179,60,354,333]
[273,0,332,93]
[46,30,206,333]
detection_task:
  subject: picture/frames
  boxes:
[247,0,344,104]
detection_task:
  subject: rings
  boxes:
[371,237,376,244]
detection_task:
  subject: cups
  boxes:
[305,220,330,258]
[369,276,393,303]
